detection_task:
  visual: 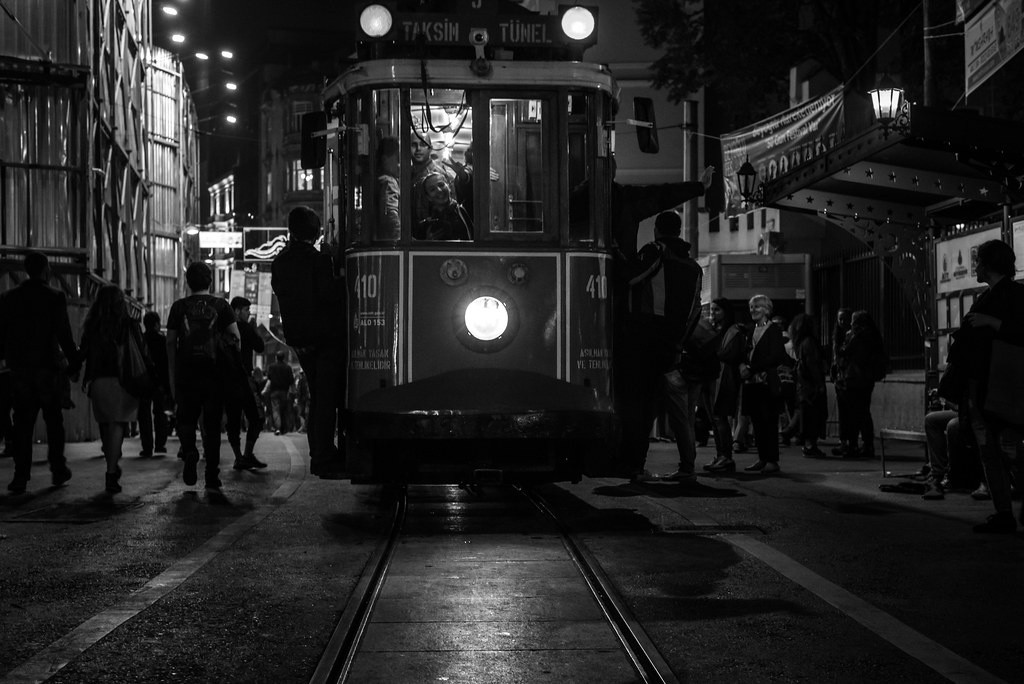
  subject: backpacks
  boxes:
[175,295,221,375]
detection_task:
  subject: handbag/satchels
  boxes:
[130,367,171,408]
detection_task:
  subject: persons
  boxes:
[167,125,1023,544]
[67,283,148,504]
[0,360,18,461]
[135,310,172,459]
[0,248,79,493]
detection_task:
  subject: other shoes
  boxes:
[972,512,1017,534]
[843,447,860,458]
[745,459,765,471]
[7,474,31,494]
[51,467,74,485]
[636,470,660,480]
[182,451,199,486]
[761,461,782,475]
[663,471,699,481]
[704,456,737,473]
[860,444,876,457]
[204,477,223,489]
[803,445,827,458]
[234,454,266,470]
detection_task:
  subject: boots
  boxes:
[104,465,123,496]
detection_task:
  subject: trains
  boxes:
[301,0,659,512]
